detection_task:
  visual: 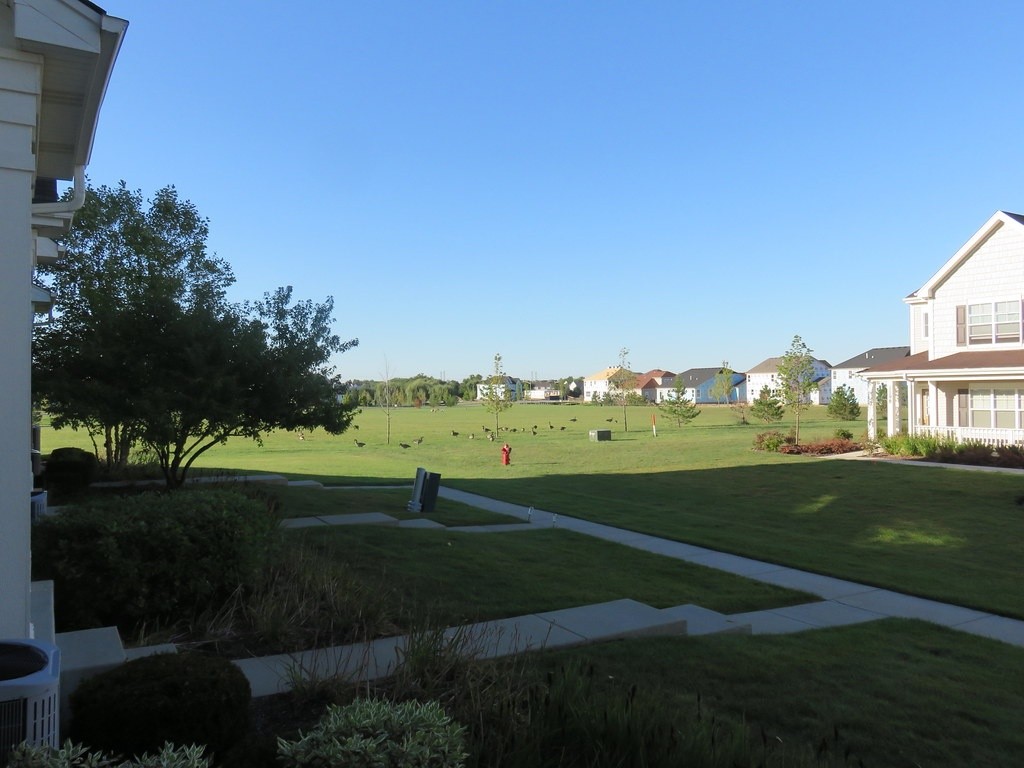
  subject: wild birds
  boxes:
[498,425,537,436]
[548,421,555,430]
[569,415,578,424]
[412,436,425,446]
[451,430,460,437]
[606,416,618,424]
[481,425,492,433]
[399,442,412,450]
[354,439,366,448]
[560,426,567,433]
[485,431,495,441]
[468,433,474,440]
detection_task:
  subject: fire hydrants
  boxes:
[501,443,512,465]
